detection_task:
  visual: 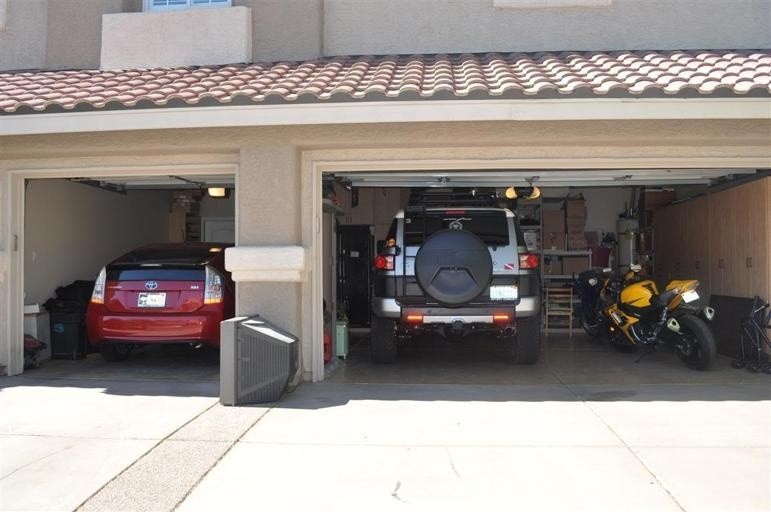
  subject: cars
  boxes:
[86,241,235,364]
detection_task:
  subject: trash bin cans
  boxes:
[42,279,101,360]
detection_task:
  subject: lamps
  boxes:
[202,175,230,198]
[503,179,544,201]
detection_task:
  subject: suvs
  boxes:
[368,205,544,366]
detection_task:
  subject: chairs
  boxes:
[545,286,573,337]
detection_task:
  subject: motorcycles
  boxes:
[590,264,719,371]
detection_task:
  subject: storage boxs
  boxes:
[542,198,589,252]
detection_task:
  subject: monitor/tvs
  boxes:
[218,313,299,406]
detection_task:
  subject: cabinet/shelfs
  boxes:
[170,212,202,241]
[336,223,374,328]
[649,173,770,355]
[542,250,592,334]
